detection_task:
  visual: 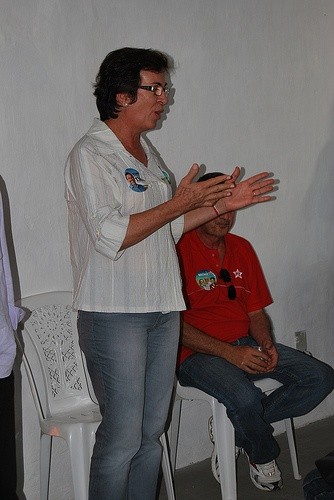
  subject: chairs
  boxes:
[16,290,176,500]
[169,378,302,500]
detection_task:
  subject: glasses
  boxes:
[220,269,236,299]
[138,85,170,96]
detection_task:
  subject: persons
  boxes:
[65,48,273,500]
[0,190,25,500]
[175,173,334,491]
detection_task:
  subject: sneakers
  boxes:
[242,448,282,492]
[208,415,240,485]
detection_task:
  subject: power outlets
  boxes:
[295,329,307,353]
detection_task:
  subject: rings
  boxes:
[254,193,255,196]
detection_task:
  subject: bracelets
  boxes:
[213,205,219,216]
